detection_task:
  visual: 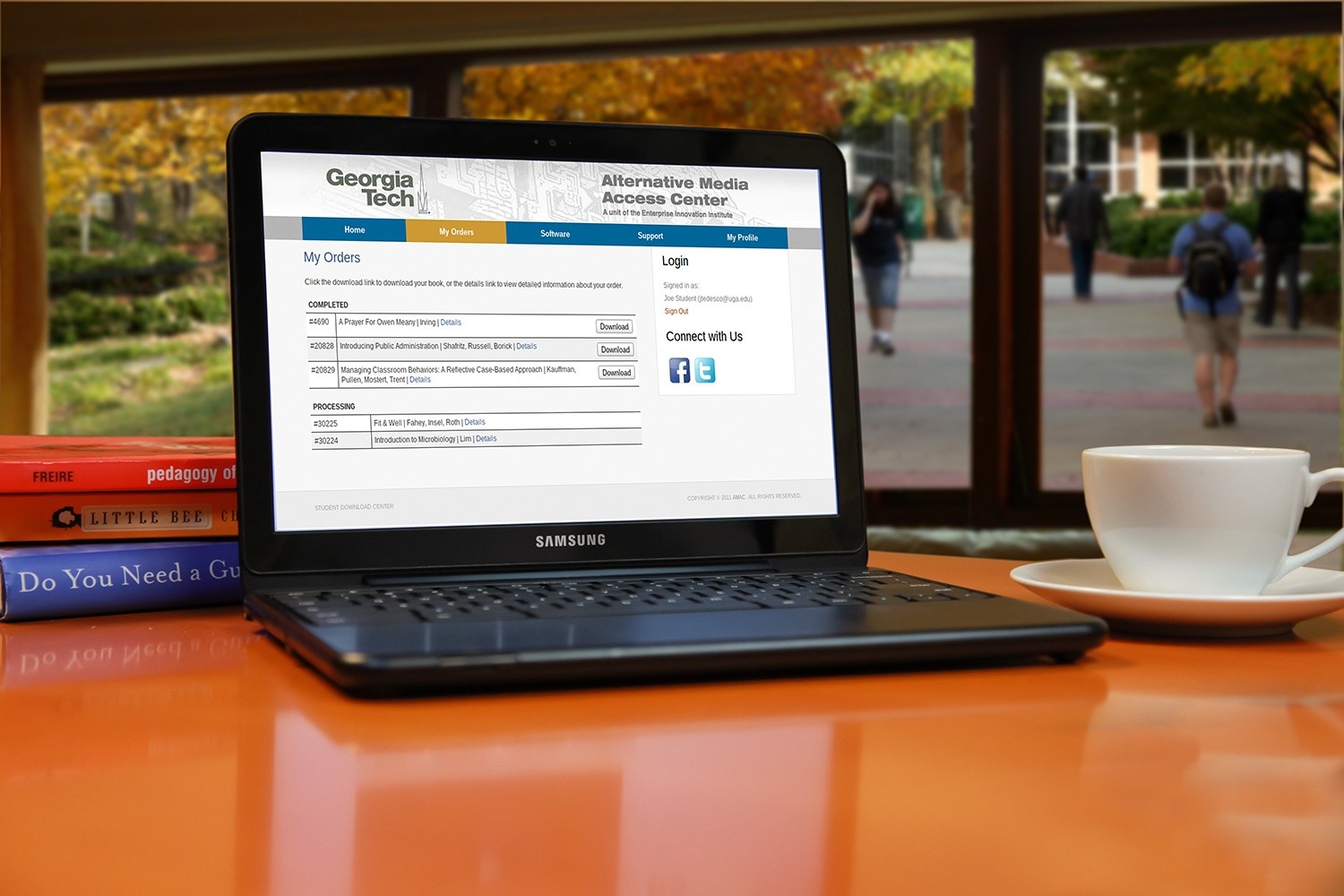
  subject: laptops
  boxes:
[222,112,1106,693]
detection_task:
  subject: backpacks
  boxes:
[1174,219,1241,323]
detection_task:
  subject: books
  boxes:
[0,435,248,621]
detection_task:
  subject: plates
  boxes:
[1009,558,1344,637]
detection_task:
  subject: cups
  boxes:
[1081,443,1344,591]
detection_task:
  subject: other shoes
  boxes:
[868,336,896,358]
[1253,313,1272,325]
[1204,416,1217,430]
[1287,321,1302,336]
[1219,399,1237,424]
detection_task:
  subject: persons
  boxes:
[846,176,914,358]
[1042,164,1111,299]
[1249,164,1306,330]
[1167,185,1259,431]
